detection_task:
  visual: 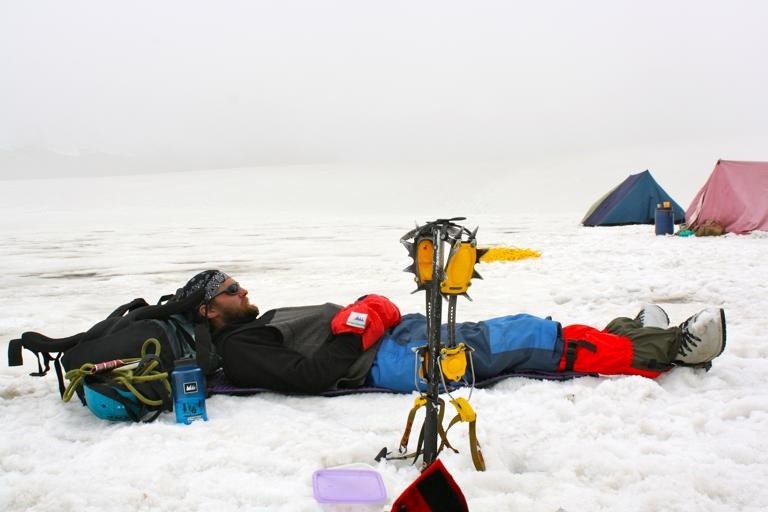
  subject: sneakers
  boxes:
[635,305,669,330]
[669,307,726,372]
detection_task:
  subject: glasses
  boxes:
[205,281,240,316]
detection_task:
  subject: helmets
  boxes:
[82,375,150,422]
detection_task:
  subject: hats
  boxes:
[176,270,230,313]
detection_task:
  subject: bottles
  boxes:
[170,356,208,425]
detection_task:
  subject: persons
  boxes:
[169,269,728,396]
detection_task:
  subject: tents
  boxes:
[680,158,768,236]
[581,168,686,225]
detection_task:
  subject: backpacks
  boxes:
[61,294,222,405]
